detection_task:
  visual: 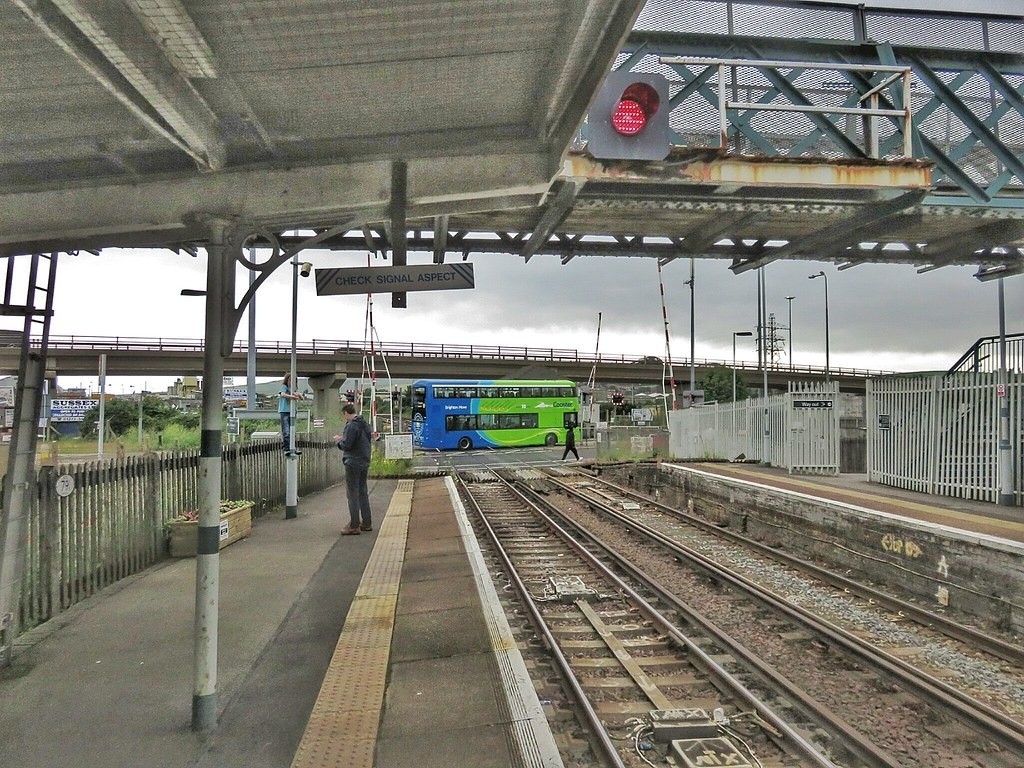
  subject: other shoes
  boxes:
[284,449,302,456]
[561,460,566,463]
[577,457,583,462]
[341,526,373,535]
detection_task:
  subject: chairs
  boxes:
[431,388,576,430]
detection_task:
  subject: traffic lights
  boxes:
[581,69,671,161]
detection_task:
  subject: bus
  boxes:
[410,378,582,450]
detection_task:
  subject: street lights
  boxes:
[808,271,829,384]
[784,296,797,371]
[732,332,753,448]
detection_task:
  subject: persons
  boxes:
[448,417,538,429]
[335,404,373,535]
[278,373,304,455]
[438,389,567,398]
[568,417,574,427]
[562,427,584,462]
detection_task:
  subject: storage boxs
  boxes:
[169,500,255,557]
